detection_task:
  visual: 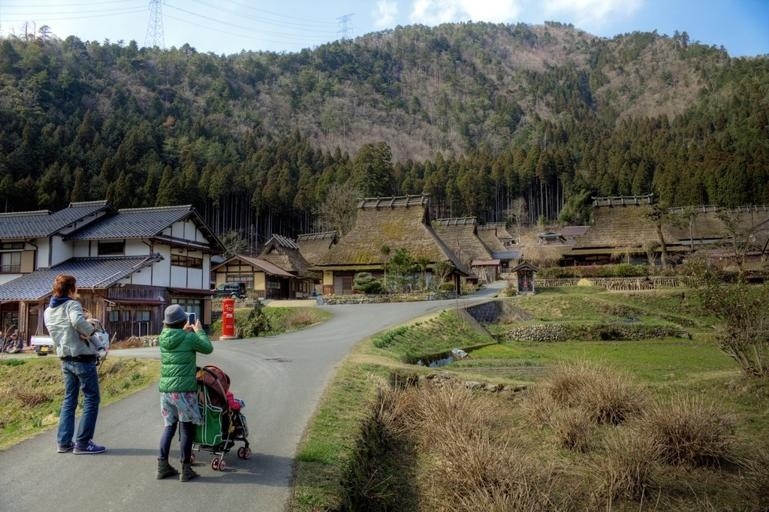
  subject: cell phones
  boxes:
[188,313,195,327]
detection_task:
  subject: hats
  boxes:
[162,303,189,325]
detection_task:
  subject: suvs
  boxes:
[210,282,247,299]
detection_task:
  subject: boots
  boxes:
[155,456,179,479]
[178,463,197,482]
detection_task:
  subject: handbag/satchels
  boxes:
[189,383,224,448]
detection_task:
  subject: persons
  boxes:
[154,304,213,483]
[225,374,243,411]
[7,333,23,354]
[43,274,105,454]
[0,332,4,352]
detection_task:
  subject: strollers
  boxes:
[188,366,252,471]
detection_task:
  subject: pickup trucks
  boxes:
[29,318,110,356]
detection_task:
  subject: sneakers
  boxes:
[57,442,75,453]
[73,441,106,455]
